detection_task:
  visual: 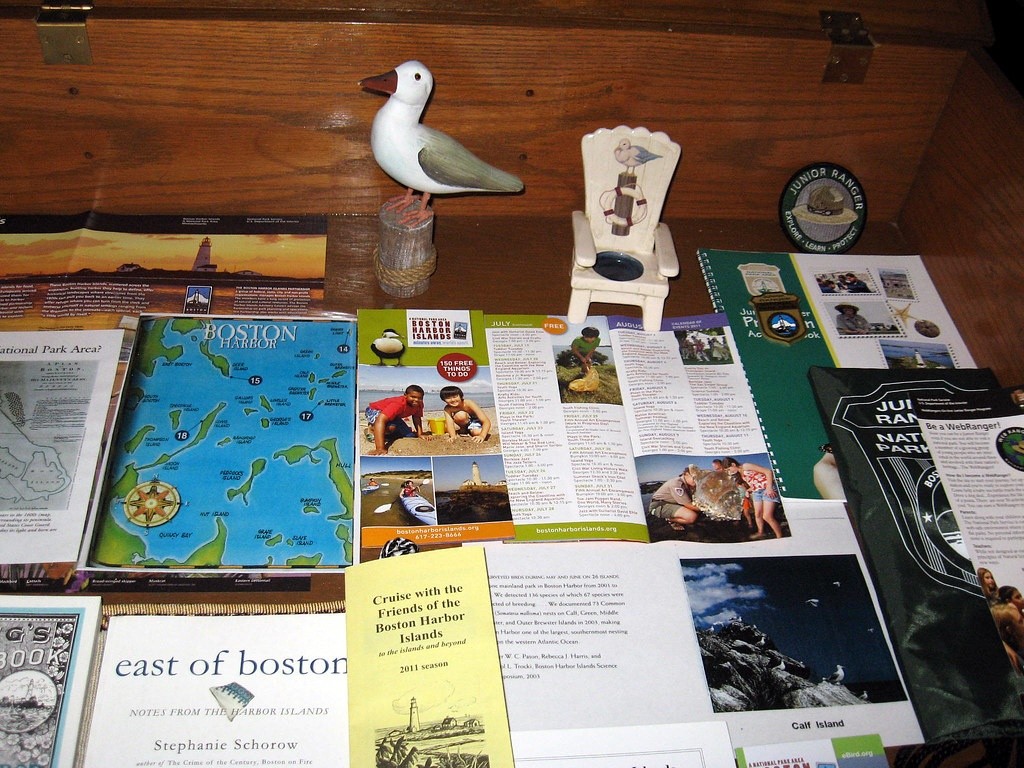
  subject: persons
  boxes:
[674,322,734,365]
[399,480,416,499]
[835,302,871,335]
[998,586,1024,618]
[732,478,754,529]
[440,386,490,443]
[816,277,823,285]
[837,275,848,289]
[844,273,871,293]
[571,327,601,365]
[648,463,704,531]
[712,459,725,473]
[977,567,1000,604]
[989,606,1023,674]
[821,281,837,294]
[365,385,434,455]
[369,478,379,486]
[722,457,783,539]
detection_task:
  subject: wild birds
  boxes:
[370,329,406,367]
[357,60,525,229]
[704,581,875,700]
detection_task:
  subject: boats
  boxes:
[361,484,379,496]
[400,492,437,525]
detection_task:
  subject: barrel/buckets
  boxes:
[427,418,446,436]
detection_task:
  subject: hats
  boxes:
[688,464,702,481]
[834,303,860,312]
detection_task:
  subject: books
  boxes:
[697,245,979,499]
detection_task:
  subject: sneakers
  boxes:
[363,428,377,445]
